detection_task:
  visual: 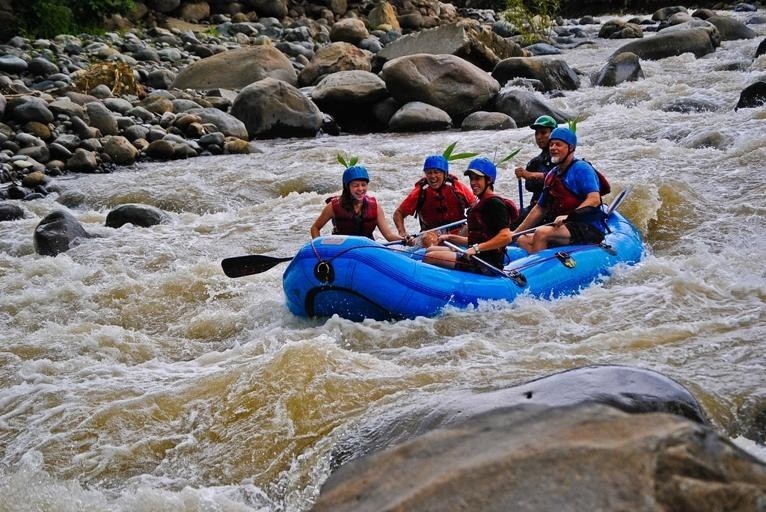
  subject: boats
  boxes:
[284,202,645,320]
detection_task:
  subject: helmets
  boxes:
[423,155,449,174]
[530,114,558,131]
[548,126,577,147]
[468,157,497,183]
[343,166,370,185]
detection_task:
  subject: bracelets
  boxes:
[472,243,480,254]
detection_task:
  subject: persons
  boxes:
[392,155,477,246]
[511,127,611,254]
[514,115,558,221]
[423,157,520,274]
[311,165,416,246]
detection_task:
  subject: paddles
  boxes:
[442,238,529,288]
[221,233,408,278]
